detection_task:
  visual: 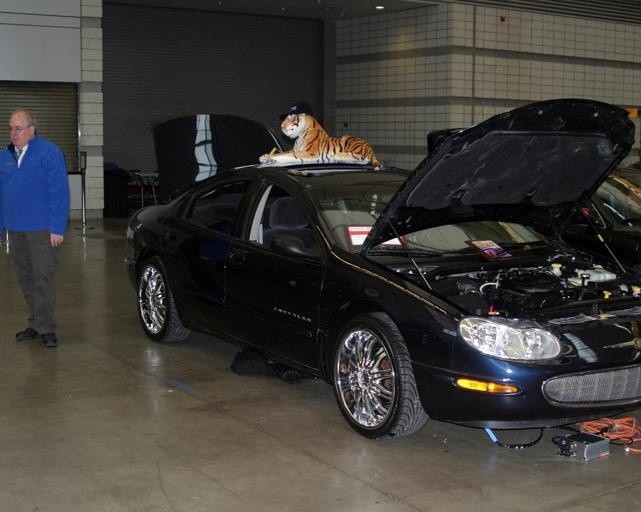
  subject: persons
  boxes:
[0,108,70,348]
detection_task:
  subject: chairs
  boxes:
[263,195,319,248]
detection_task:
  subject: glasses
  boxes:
[9,125,30,131]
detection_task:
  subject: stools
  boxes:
[134,171,160,208]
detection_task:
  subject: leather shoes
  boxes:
[15,328,39,341]
[39,333,58,348]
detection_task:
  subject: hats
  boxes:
[280,103,313,118]
